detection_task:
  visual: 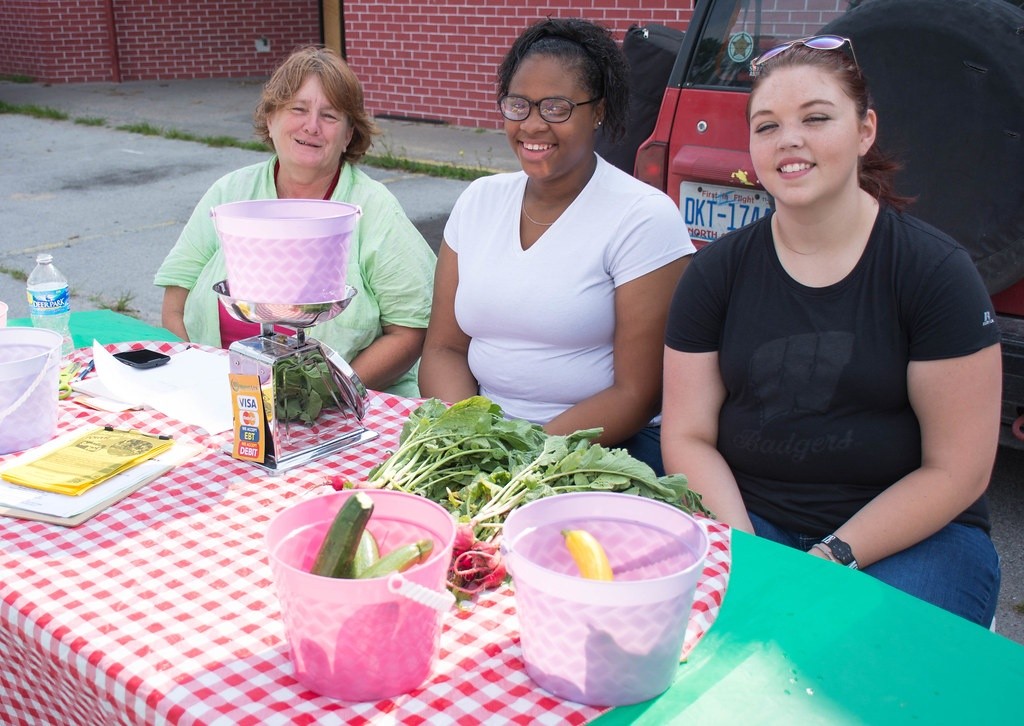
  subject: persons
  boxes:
[659,33,1005,626]
[417,20,696,475]
[150,48,438,398]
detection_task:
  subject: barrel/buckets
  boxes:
[263,491,456,703]
[502,493,711,706]
[0,325,64,454]
[0,301,8,327]
[209,198,365,304]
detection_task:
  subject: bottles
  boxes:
[28,254,75,370]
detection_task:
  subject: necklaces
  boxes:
[522,199,553,229]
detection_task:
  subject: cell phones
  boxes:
[113,349,171,370]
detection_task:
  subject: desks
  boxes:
[0,307,1024,725]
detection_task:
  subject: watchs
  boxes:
[821,534,859,572]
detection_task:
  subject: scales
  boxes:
[212,278,381,474]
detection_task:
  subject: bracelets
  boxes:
[812,543,836,562]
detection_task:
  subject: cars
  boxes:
[631,0,1024,461]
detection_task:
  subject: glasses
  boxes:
[497,95,598,124]
[752,34,862,80]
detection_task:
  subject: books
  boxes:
[0,424,203,527]
